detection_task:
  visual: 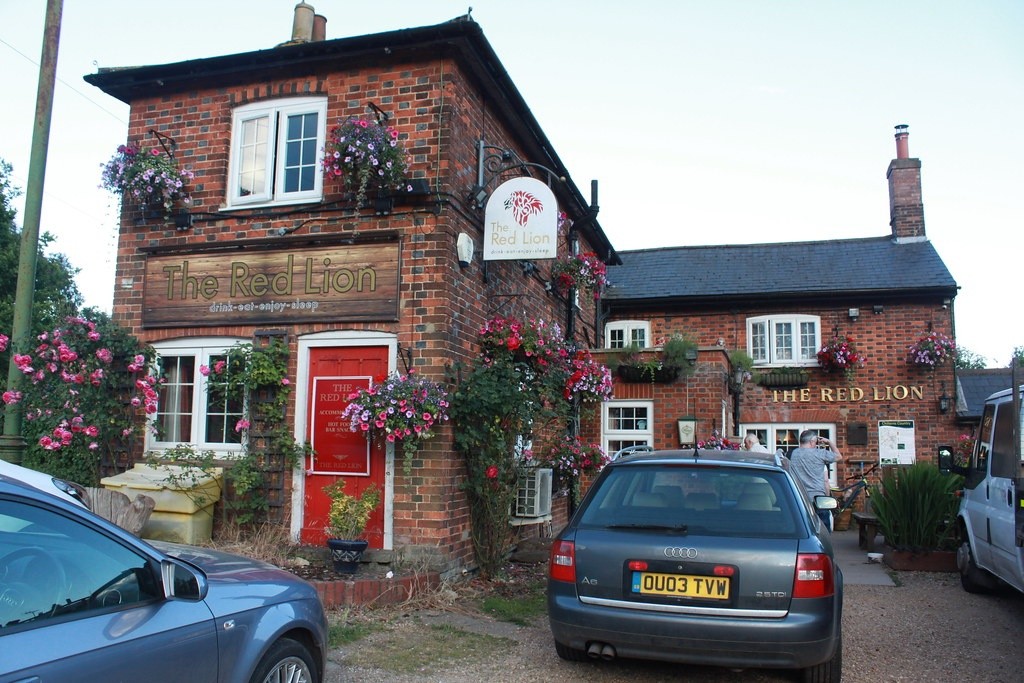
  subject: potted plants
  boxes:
[320,480,382,576]
[833,495,853,531]
[865,459,971,572]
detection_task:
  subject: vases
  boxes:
[516,468,553,517]
[617,366,684,384]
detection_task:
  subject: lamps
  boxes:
[466,185,488,204]
[728,361,745,393]
[849,308,860,322]
[939,391,953,415]
[686,349,697,366]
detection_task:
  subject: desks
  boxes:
[852,512,884,552]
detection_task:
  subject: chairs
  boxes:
[630,482,777,510]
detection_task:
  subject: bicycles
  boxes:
[830,463,879,521]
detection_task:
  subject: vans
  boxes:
[937,384,1023,595]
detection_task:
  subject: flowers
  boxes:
[908,330,956,368]
[551,210,611,302]
[318,109,413,246]
[340,315,614,493]
[817,335,867,378]
[99,140,196,228]
[959,434,977,461]
[603,336,698,384]
[676,429,741,452]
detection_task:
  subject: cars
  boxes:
[0,481,329,683]
[548,444,844,683]
[0,457,93,536]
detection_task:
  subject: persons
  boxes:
[744,434,768,483]
[791,430,842,532]
[824,465,829,496]
